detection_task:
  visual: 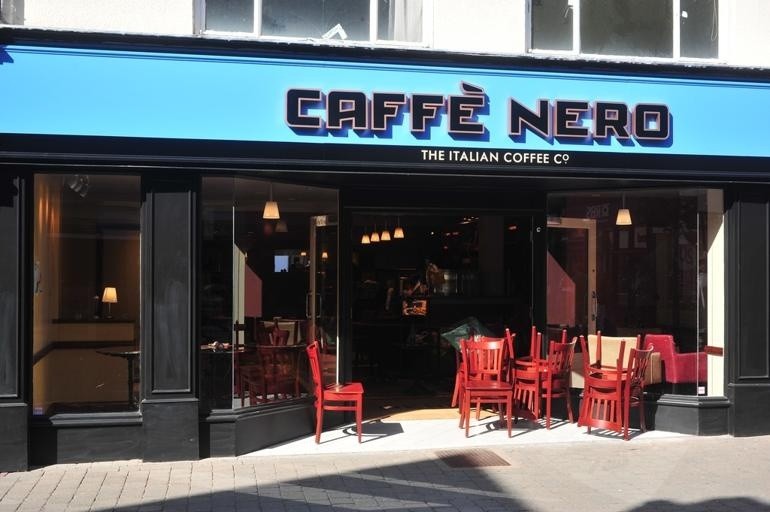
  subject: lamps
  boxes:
[262,180,289,233]
[67,174,89,197]
[361,215,405,244]
[614,193,633,226]
[102,286,118,319]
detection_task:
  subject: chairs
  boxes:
[305,341,365,444]
[198,315,336,405]
[452,325,707,439]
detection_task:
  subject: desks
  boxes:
[97,347,233,409]
[353,321,441,378]
